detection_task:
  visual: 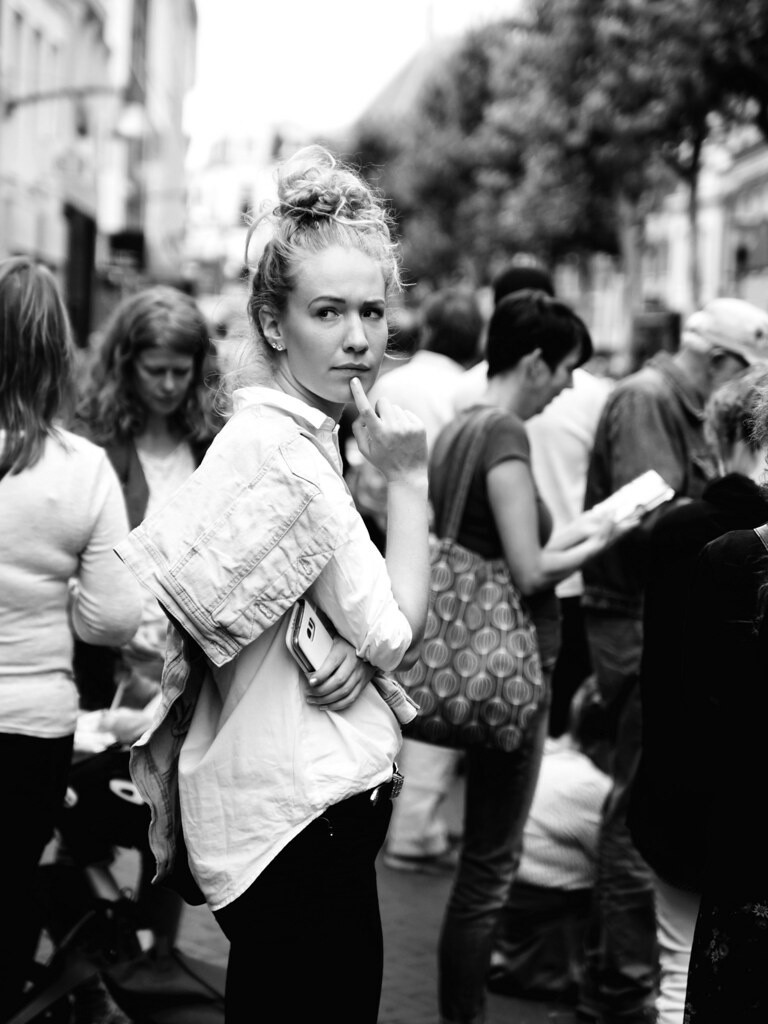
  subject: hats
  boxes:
[687,298,767,365]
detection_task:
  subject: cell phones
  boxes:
[284,598,332,689]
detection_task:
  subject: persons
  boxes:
[3,254,145,1024]
[70,279,238,1024]
[326,243,767,1024]
[176,148,432,1024]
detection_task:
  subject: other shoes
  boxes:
[384,849,458,877]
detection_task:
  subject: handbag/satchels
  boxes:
[393,408,544,751]
